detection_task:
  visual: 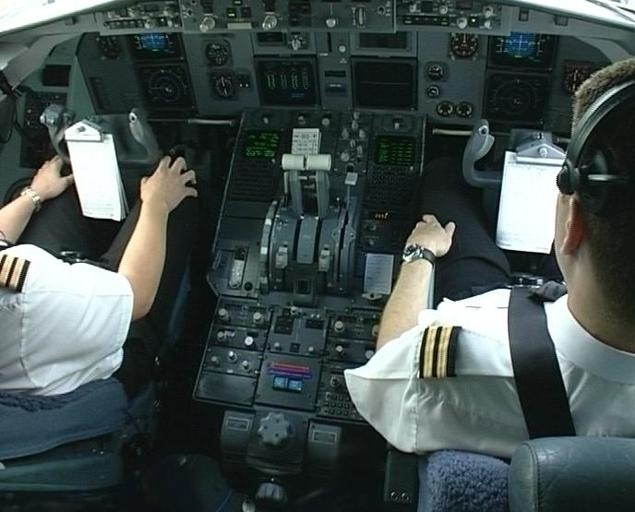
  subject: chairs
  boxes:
[387,435,635,511]
[2,375,133,507]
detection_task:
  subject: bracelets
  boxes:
[20,185,42,213]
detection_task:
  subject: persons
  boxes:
[0,156,199,397]
[342,59,635,457]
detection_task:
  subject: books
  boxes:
[67,133,130,222]
[495,150,567,254]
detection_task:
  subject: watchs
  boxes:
[400,243,437,266]
